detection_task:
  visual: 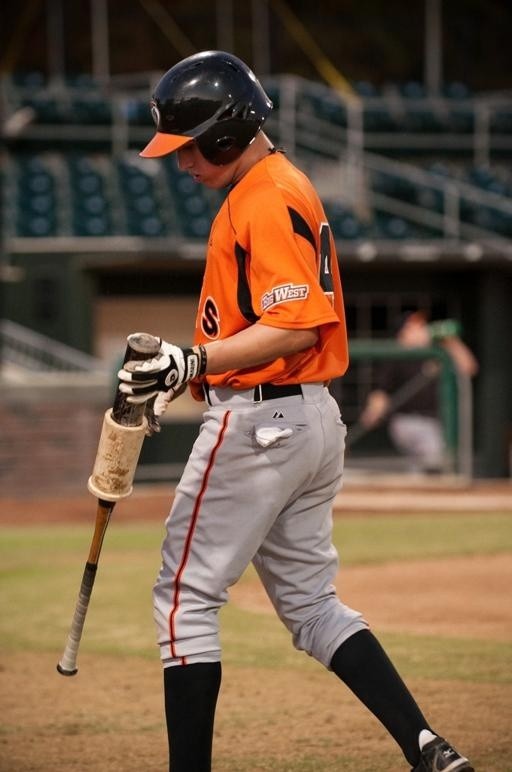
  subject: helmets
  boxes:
[134,49,274,167]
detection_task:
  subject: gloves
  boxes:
[116,334,201,419]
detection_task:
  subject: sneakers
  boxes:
[405,735,476,771]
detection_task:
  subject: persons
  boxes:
[363,310,480,459]
[117,49,481,771]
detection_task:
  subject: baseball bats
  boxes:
[55,331,158,677]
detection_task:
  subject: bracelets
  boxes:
[198,345,207,376]
